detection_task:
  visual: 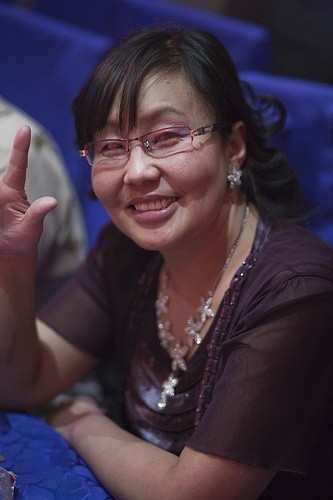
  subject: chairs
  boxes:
[0,0,333,249]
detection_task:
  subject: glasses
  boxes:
[79,123,228,168]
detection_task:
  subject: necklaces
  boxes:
[155,194,250,409]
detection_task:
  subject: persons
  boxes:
[0,23,333,500]
[0,95,102,408]
